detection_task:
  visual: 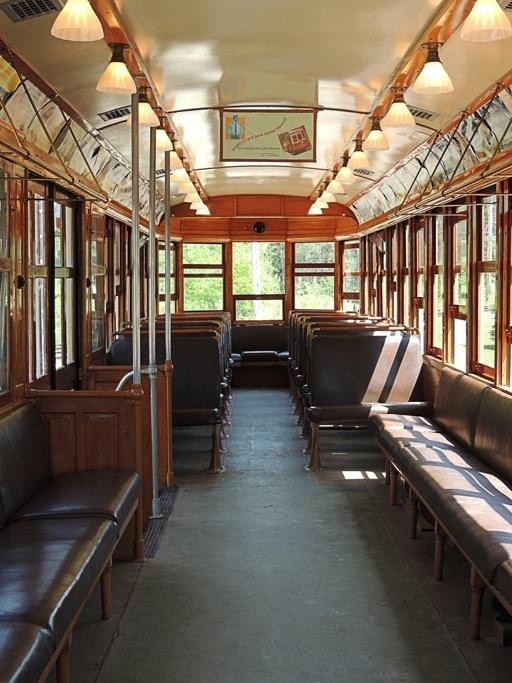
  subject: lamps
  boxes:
[414,61,454,94]
[325,180,346,194]
[461,0,510,43]
[347,151,370,169]
[335,167,356,182]
[313,195,329,208]
[320,190,336,203]
[45,0,214,217]
[363,130,390,152]
[307,201,322,216]
[380,102,415,128]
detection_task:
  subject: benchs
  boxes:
[369,362,512,657]
[0,398,139,683]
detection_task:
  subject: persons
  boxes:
[225,113,248,142]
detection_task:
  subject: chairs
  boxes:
[232,324,289,388]
[108,310,232,474]
[288,310,435,472]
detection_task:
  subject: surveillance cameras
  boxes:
[255,222,265,233]
